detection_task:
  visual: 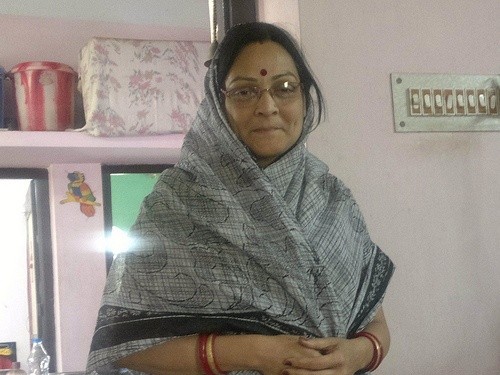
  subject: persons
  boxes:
[83,21,395,375]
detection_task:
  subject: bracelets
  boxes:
[356,330,383,375]
[197,328,222,375]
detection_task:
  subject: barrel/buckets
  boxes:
[8,62,78,131]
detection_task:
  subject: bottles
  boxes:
[27,338,50,375]
[6,362,27,375]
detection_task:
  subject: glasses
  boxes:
[220,81,302,102]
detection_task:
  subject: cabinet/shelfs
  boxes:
[0,15,211,375]
